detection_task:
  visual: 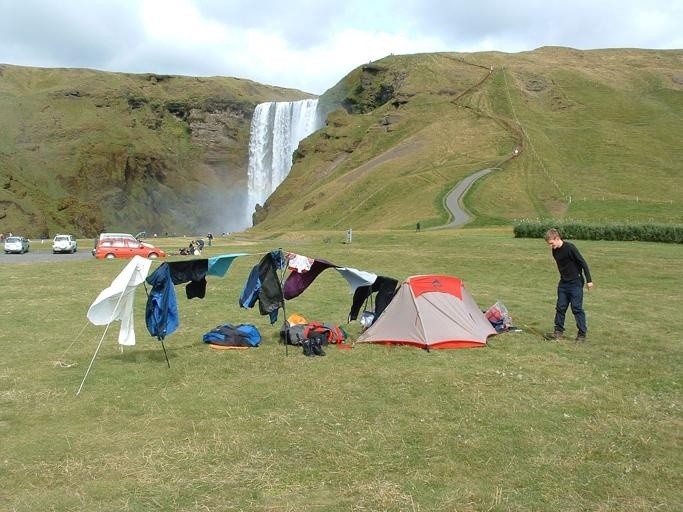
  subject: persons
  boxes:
[545,229,593,342]
[180,232,213,255]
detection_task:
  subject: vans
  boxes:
[94,231,154,249]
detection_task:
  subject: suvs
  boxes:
[90,238,165,260]
[51,235,76,254]
[3,236,28,254]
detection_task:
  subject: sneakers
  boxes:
[545,331,564,340]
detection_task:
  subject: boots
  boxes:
[302,337,325,357]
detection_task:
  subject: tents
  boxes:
[355,274,499,353]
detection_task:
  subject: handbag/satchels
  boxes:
[202,323,261,346]
[280,321,346,346]
